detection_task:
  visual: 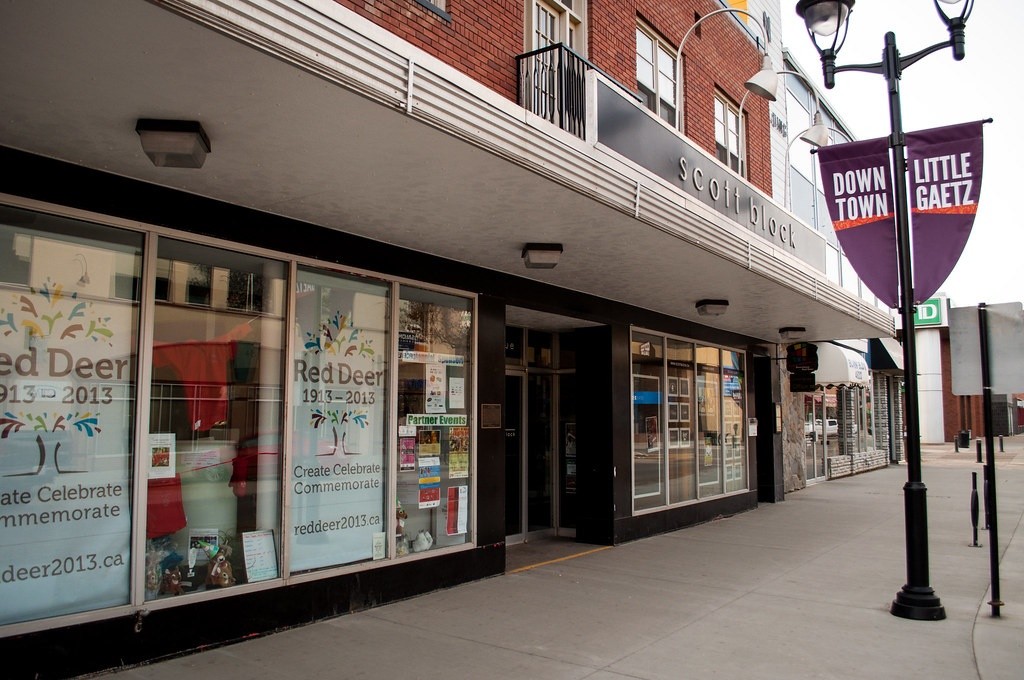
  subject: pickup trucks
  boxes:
[805,419,838,437]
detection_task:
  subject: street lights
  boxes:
[796,0,974,621]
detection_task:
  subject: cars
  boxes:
[867,425,872,435]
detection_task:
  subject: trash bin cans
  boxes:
[959,430,969,448]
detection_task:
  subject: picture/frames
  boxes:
[679,378,690,397]
[679,402,690,422]
[668,402,679,422]
[669,428,679,449]
[725,401,742,482]
[680,427,691,448]
[667,376,678,397]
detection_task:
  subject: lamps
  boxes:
[779,326,806,339]
[738,70,828,175]
[135,117,212,169]
[521,243,563,270]
[695,298,729,315]
[675,7,779,131]
